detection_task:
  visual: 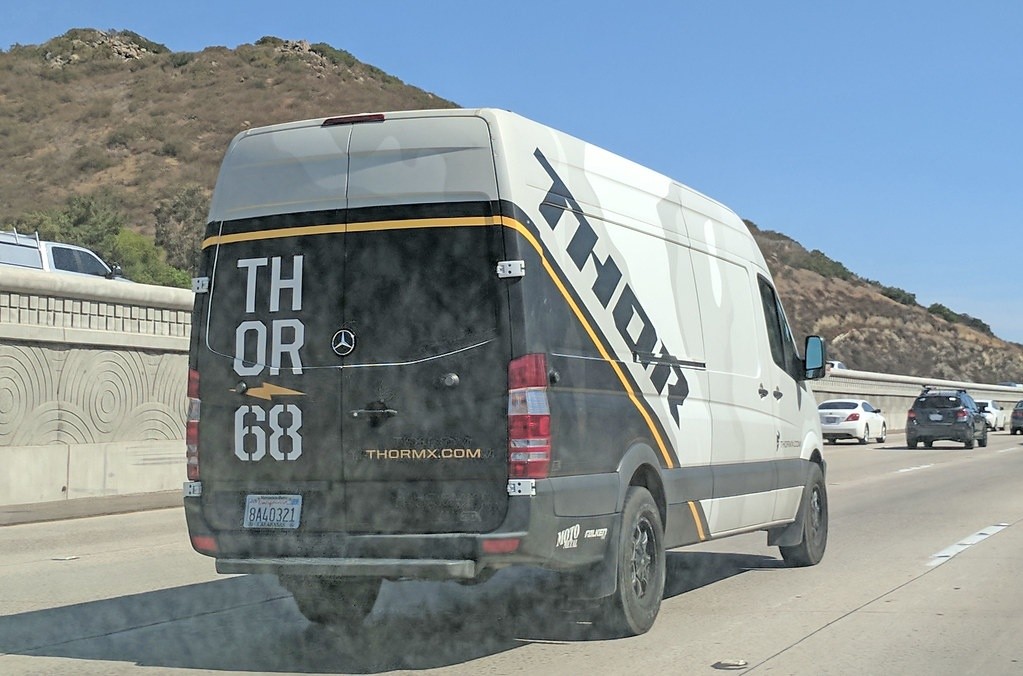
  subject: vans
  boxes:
[182,108,829,638]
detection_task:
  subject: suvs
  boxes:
[906,387,987,449]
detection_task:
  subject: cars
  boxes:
[826,360,846,371]
[817,399,887,445]
[974,399,1006,432]
[1009,401,1023,435]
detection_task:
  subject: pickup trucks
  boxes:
[0,227,142,285]
